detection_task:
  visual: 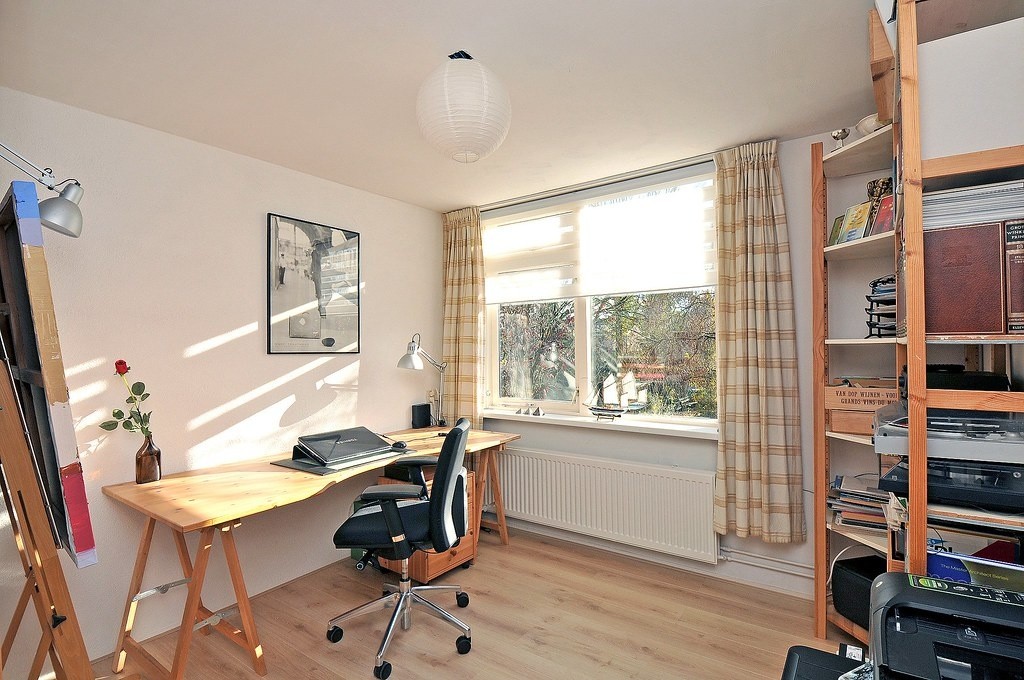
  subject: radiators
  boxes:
[475,444,725,566]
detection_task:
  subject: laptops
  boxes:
[297,426,393,465]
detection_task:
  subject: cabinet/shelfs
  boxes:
[377,471,477,587]
[812,1,1024,652]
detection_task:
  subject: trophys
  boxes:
[830,127,851,152]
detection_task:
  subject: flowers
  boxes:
[99,358,153,438]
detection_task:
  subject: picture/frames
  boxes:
[267,212,360,354]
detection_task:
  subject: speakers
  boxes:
[832,554,887,631]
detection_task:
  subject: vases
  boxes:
[134,435,162,485]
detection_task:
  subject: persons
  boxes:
[310,241,333,317]
[278,253,286,285]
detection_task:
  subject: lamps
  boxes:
[0,143,84,240]
[414,48,512,163]
[396,333,449,427]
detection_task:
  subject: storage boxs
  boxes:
[825,374,898,435]
[350,499,388,572]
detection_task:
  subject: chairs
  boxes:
[325,417,472,680]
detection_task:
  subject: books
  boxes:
[825,470,908,537]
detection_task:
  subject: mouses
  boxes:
[392,441,407,448]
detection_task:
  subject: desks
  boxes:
[101,423,521,680]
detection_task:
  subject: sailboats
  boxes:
[584,374,628,417]
[622,372,647,411]
